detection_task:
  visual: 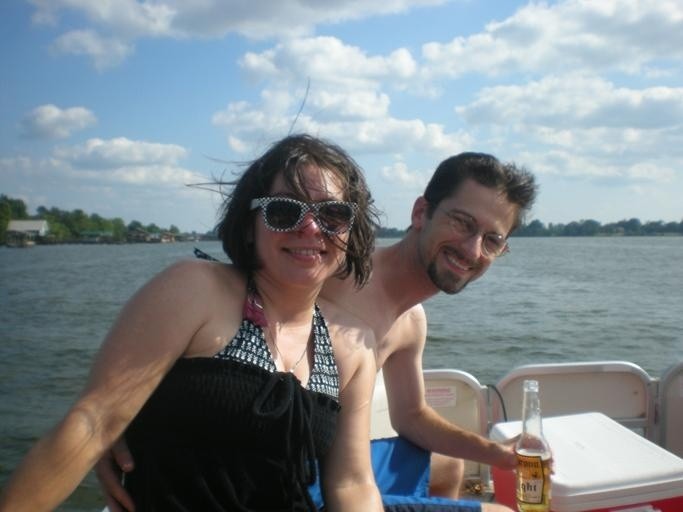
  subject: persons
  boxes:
[93,151,558,512]
[0,132,385,512]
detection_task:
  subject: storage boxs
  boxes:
[489,412,683,512]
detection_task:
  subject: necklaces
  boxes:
[264,320,306,373]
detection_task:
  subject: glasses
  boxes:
[247,196,360,235]
[431,201,512,258]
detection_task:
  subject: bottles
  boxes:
[514,379,553,512]
